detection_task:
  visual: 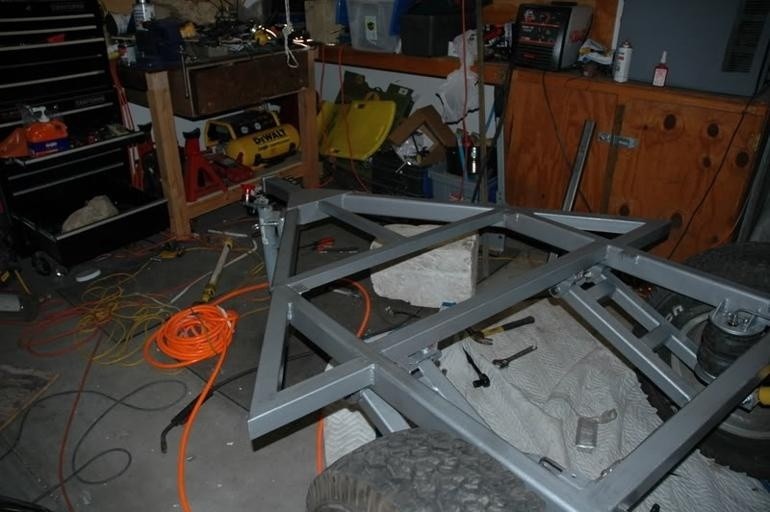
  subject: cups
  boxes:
[468,145,480,174]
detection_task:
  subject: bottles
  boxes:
[612,39,633,84]
[0,291,36,323]
[651,50,669,87]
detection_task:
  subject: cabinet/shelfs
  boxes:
[114,41,318,243]
[502,67,770,277]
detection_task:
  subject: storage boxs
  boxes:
[339,1,485,54]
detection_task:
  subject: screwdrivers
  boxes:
[299,235,335,250]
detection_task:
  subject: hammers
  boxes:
[465,315,535,345]
